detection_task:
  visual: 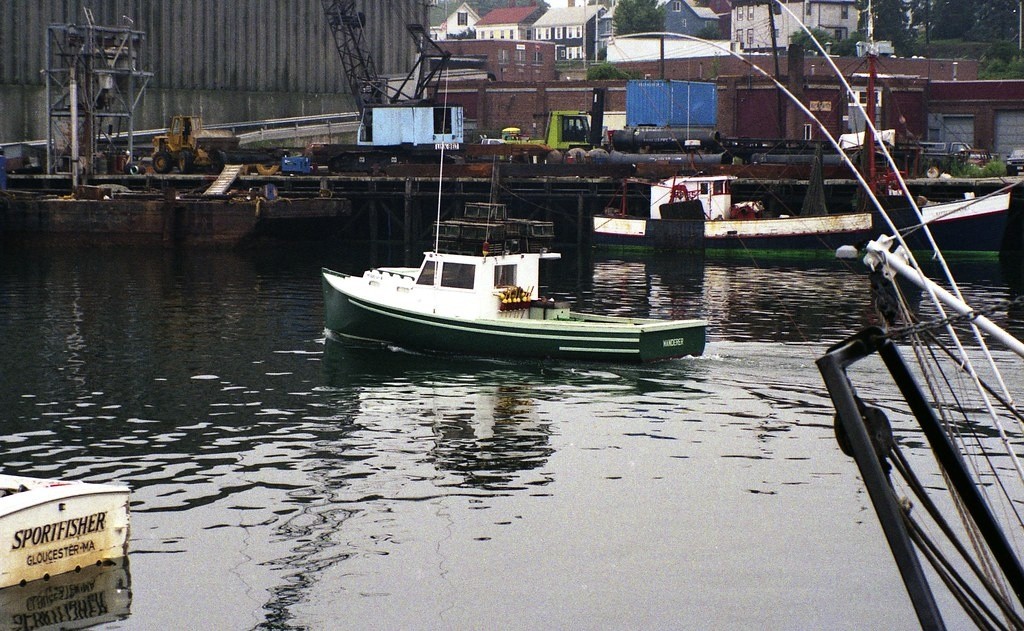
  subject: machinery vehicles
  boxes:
[150,114,242,175]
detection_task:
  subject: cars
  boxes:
[1005,149,1023,177]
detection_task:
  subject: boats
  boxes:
[321,65,710,366]
[591,1,1010,264]
[0,473,130,591]
